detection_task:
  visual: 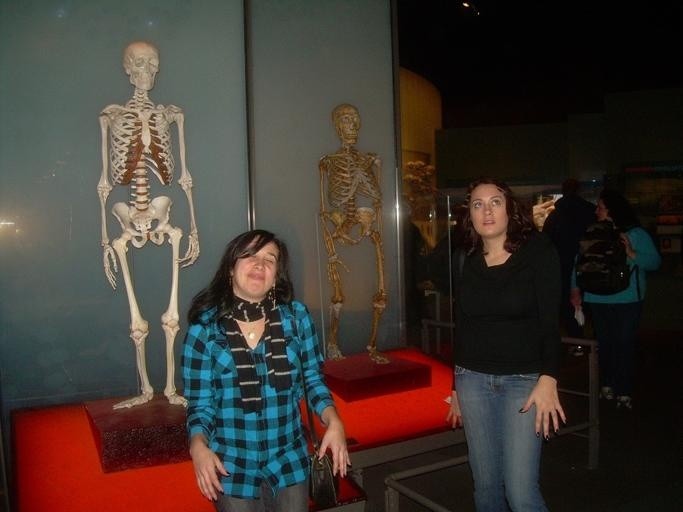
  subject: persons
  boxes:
[542,179,599,357]
[570,189,663,409]
[178,229,350,512]
[445,175,573,511]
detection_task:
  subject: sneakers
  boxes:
[616,394,634,411]
[598,386,614,401]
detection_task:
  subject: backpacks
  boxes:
[572,218,638,296]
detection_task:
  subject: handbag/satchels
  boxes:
[309,446,338,503]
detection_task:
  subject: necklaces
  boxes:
[244,321,259,339]
